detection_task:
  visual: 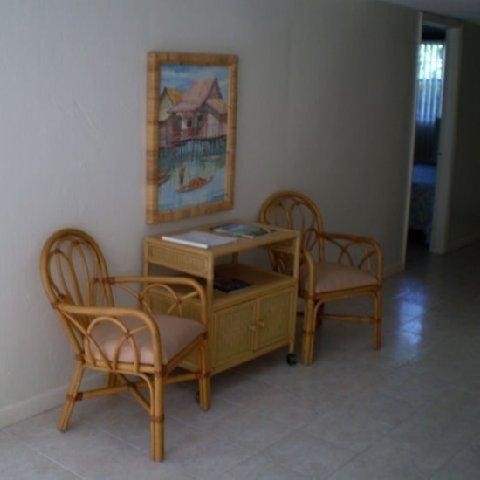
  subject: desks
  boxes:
[149,219,299,409]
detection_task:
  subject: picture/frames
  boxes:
[145,50,237,223]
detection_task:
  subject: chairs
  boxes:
[250,188,385,367]
[33,228,213,463]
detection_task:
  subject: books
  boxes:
[209,221,278,240]
[198,273,253,294]
[162,229,239,250]
